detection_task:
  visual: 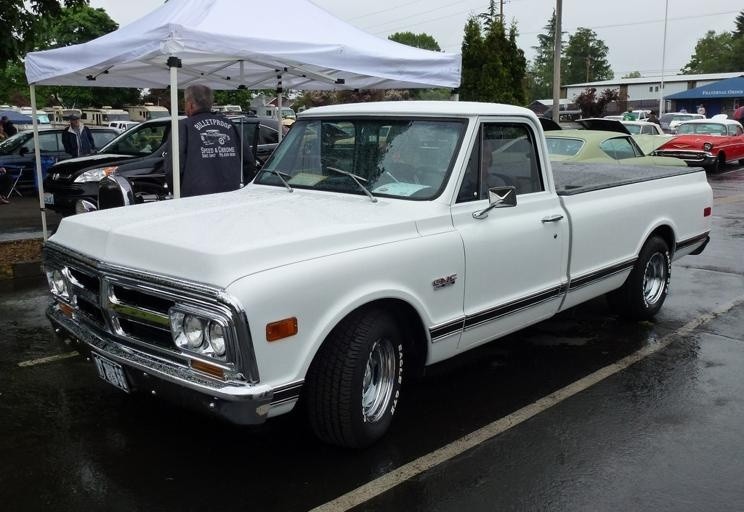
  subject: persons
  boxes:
[622,106,634,121]
[0,116,17,138]
[734,100,744,136]
[62,115,97,157]
[162,84,255,198]
[696,103,706,116]
[647,111,660,127]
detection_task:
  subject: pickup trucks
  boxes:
[36,99,714,454]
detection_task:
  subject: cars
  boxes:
[0,101,398,220]
[491,109,728,171]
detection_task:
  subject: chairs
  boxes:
[462,137,507,199]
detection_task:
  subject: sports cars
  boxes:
[649,118,744,174]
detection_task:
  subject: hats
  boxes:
[71,112,80,120]
